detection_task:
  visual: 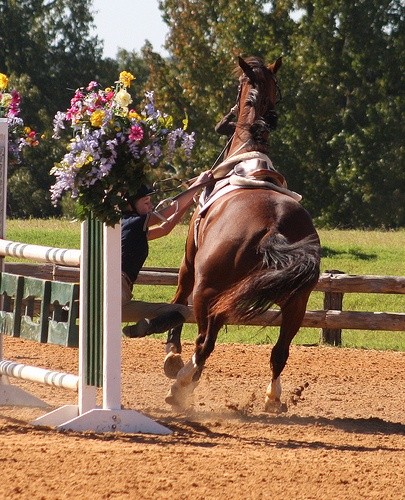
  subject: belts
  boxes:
[121,273,133,292]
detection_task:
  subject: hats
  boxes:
[122,186,156,207]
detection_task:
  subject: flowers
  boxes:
[46,70,198,229]
[0,72,40,168]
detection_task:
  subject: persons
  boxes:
[121,169,214,339]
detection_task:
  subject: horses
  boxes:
[162,51,322,414]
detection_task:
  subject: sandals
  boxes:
[123,310,187,339]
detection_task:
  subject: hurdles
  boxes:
[0,119,173,434]
